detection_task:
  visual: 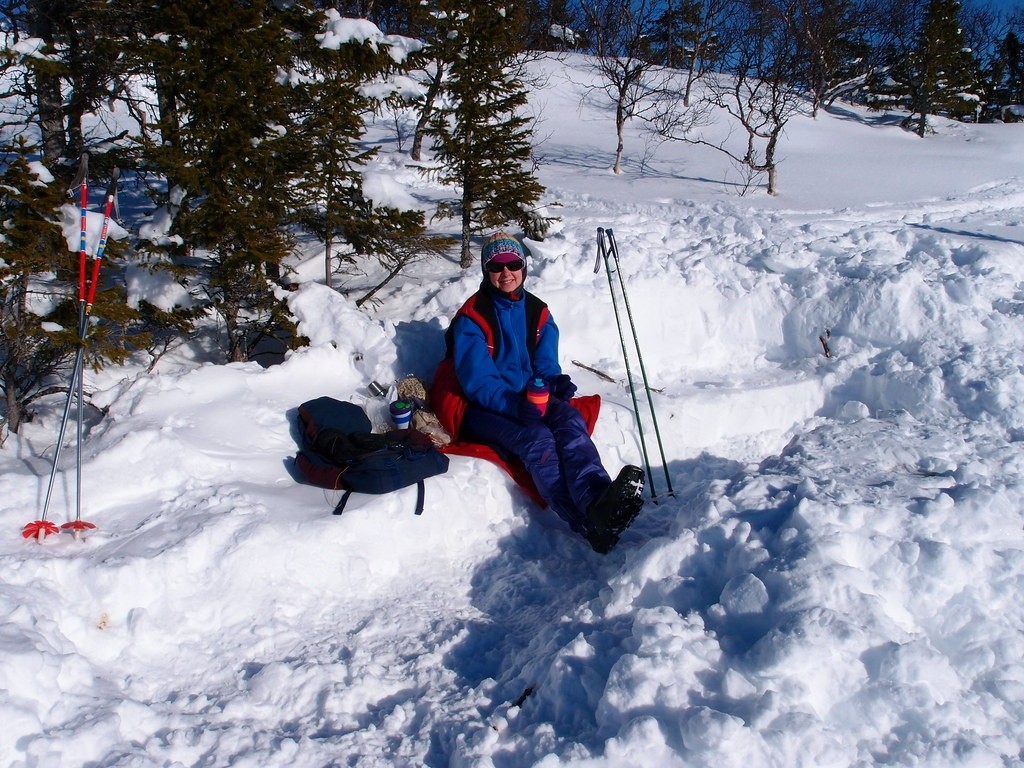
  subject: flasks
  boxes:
[367,381,388,399]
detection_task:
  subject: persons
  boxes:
[443,231,646,554]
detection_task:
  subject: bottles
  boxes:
[389,399,411,430]
[526,377,550,418]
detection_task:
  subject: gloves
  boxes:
[517,386,542,428]
[556,374,577,403]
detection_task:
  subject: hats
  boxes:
[481,233,526,274]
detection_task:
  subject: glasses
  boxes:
[486,260,523,273]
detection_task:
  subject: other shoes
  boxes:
[587,499,644,555]
[588,465,645,527]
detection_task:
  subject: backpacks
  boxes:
[295,429,449,515]
[296,396,372,444]
